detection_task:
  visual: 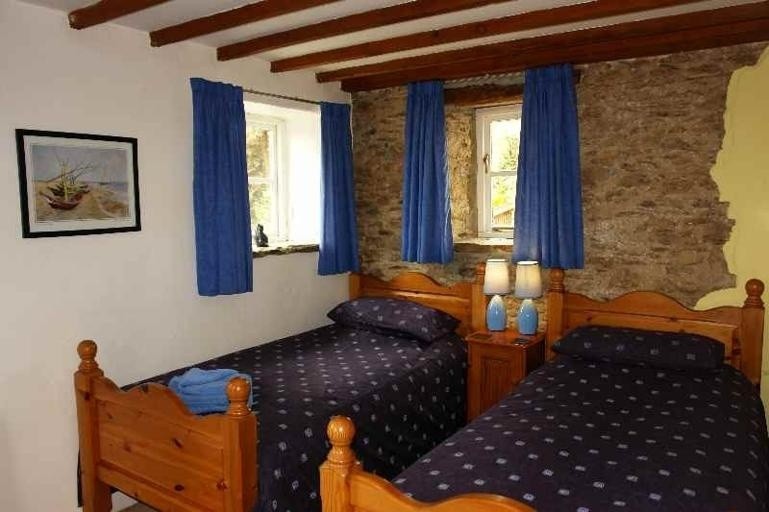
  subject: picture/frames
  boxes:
[14,127,141,239]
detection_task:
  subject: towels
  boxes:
[167,367,254,415]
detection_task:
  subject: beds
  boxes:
[73,261,491,512]
[317,266,765,512]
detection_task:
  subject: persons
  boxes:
[255,223,268,247]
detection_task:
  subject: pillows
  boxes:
[550,324,724,373]
[327,295,463,345]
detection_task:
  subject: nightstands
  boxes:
[464,328,546,425]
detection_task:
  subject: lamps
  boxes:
[482,257,543,336]
